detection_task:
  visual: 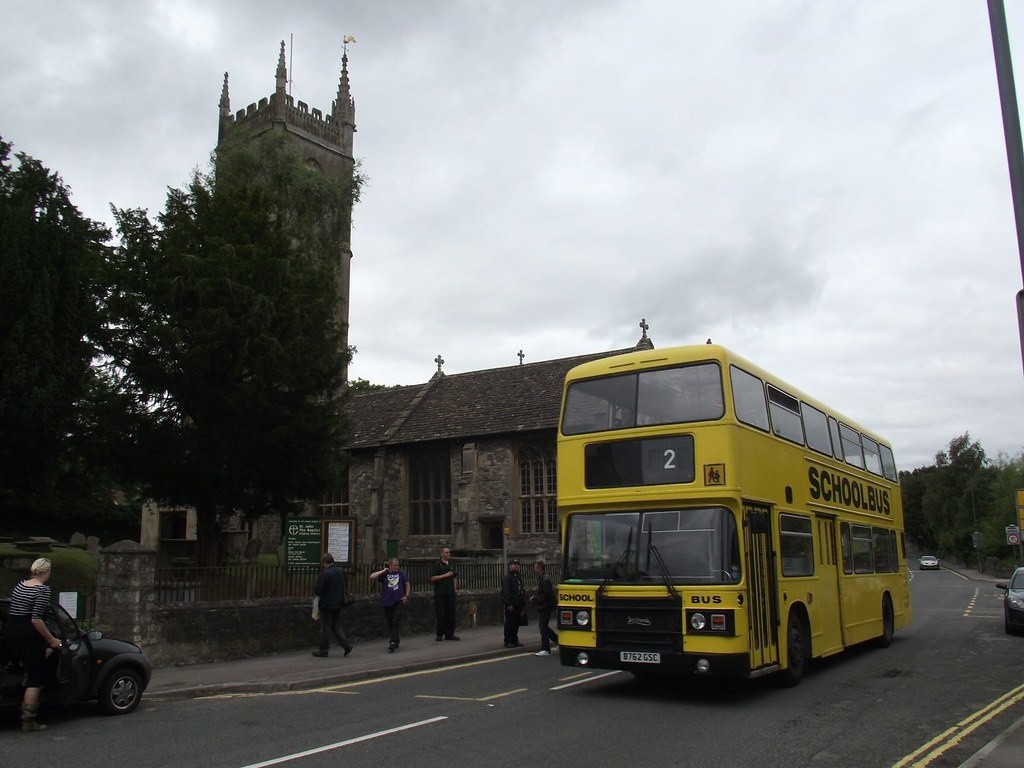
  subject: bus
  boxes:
[553,343,911,692]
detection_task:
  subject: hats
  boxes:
[508,559,520,563]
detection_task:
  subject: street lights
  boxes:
[940,463,983,573]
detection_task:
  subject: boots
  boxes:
[21,703,47,732]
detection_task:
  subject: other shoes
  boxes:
[445,635,460,640]
[514,643,524,647]
[504,643,515,648]
[535,650,552,656]
[389,641,399,650]
[436,634,442,641]
[344,643,354,657]
[312,651,328,657]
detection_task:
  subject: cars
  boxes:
[995,566,1024,636]
[0,595,152,716]
[918,555,940,570]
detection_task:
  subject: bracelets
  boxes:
[406,596,410,600]
[48,638,55,643]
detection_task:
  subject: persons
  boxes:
[369,557,411,650]
[310,554,353,657]
[530,561,560,656]
[431,547,460,641]
[7,558,62,732]
[601,527,640,570]
[501,559,525,648]
